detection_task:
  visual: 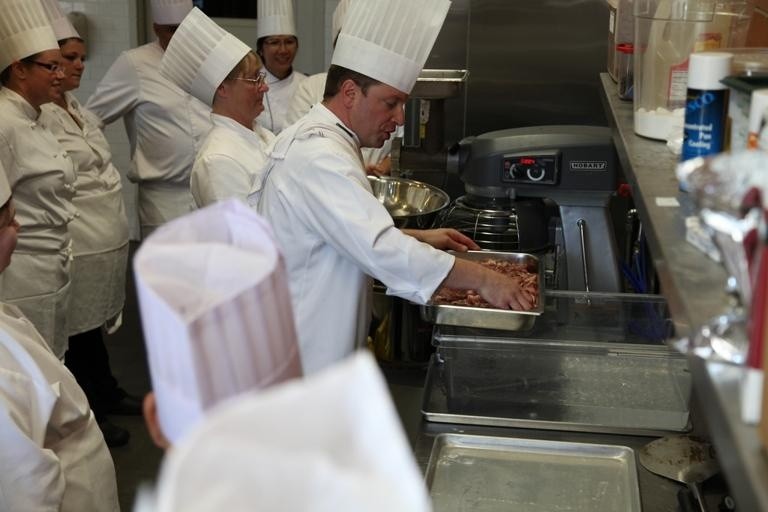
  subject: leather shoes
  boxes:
[96,417,130,447]
[101,393,143,415]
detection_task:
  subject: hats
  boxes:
[0,0,82,72]
[257,0,297,39]
[151,0,252,105]
[132,198,304,445]
[331,0,452,96]
[133,348,431,512]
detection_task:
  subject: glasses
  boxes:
[230,72,267,85]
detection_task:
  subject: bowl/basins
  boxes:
[358,176,450,228]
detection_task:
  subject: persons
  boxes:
[139,390,176,456]
[282,29,399,181]
[77,20,216,243]
[39,37,149,449]
[254,34,313,140]
[0,49,78,389]
[254,66,538,386]
[0,194,125,512]
[187,52,283,208]
